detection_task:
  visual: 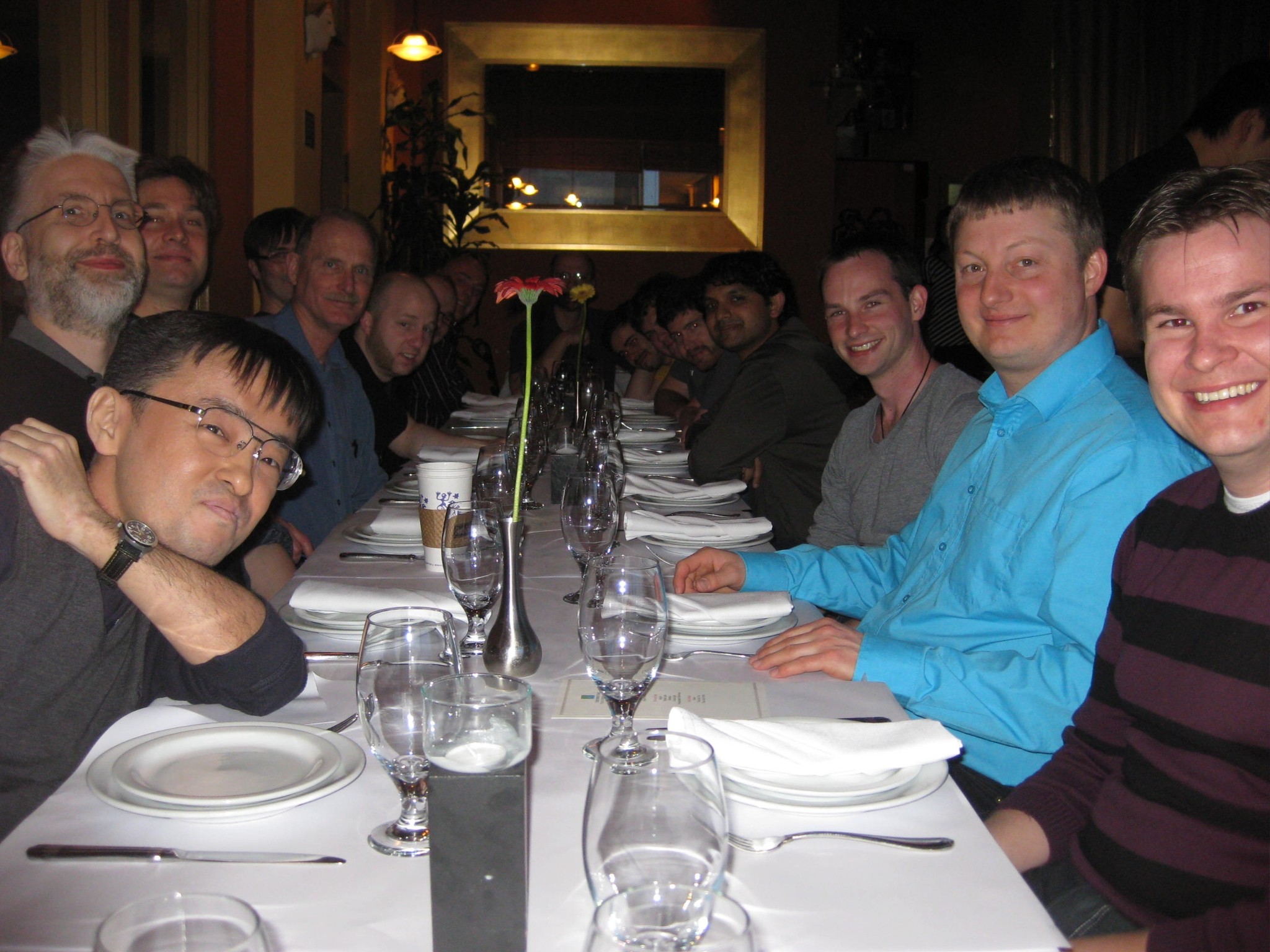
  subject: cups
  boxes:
[577,728,753,952]
[93,890,272,952]
[419,671,533,774]
[472,358,625,526]
[415,461,475,573]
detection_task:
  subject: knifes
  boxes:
[26,843,346,865]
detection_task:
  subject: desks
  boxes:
[0,391,1071,952]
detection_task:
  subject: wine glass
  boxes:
[442,500,506,658]
[355,606,465,860]
[578,554,670,773]
[559,471,618,608]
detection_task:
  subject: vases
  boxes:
[568,381,587,447]
[483,515,545,678]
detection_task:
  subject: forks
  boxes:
[327,713,359,733]
[700,819,955,854]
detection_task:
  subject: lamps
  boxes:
[386,0,442,63]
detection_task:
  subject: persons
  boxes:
[670,158,1212,824]
[243,205,313,316]
[804,235,990,552]
[509,74,1270,952]
[0,120,500,841]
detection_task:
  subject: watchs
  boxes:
[97,520,159,590]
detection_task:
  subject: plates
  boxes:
[341,522,423,548]
[617,405,798,642]
[85,721,367,822]
[721,758,950,814]
[460,417,509,423]
[383,478,419,497]
[277,602,367,639]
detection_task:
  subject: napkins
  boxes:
[617,469,748,500]
[78,668,362,760]
[285,575,493,625]
[622,447,691,464]
[599,592,794,625]
[620,397,654,415]
[416,443,480,462]
[616,429,676,441]
[450,390,524,418]
[663,703,963,779]
[371,504,500,541]
[622,509,774,542]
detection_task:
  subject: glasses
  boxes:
[120,388,306,491]
[257,249,287,264]
[15,196,148,233]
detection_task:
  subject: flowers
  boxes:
[568,284,597,381]
[494,276,566,521]
